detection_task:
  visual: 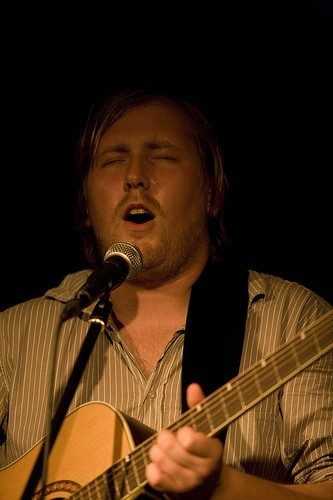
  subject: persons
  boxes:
[0,82,333,500]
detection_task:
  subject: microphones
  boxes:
[62,241,145,322]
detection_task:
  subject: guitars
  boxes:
[0,307,333,499]
